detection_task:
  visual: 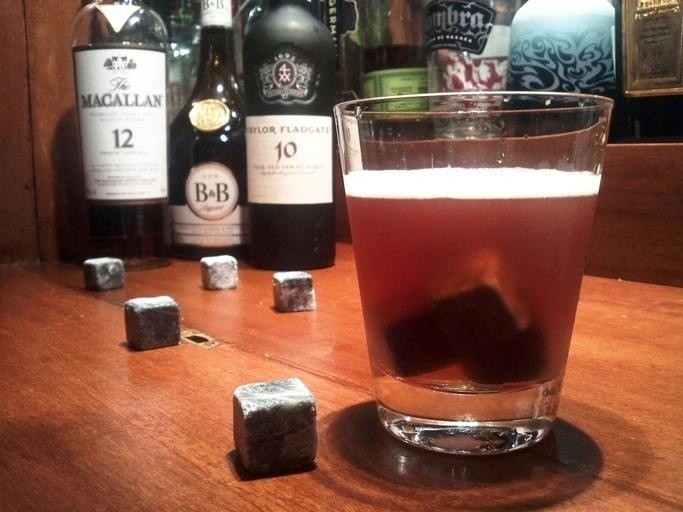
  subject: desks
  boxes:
[2,241,683,511]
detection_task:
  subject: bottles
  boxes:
[360,0,614,120]
[68,0,336,271]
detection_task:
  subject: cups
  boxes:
[331,90,612,458]
